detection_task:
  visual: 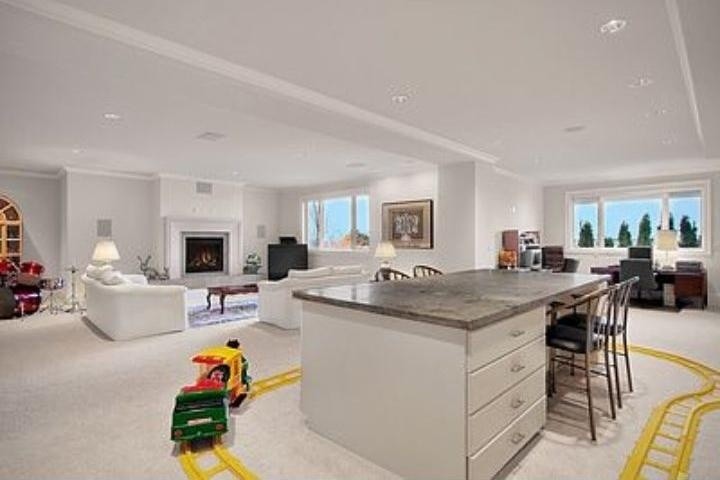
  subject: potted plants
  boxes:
[243,252,262,275]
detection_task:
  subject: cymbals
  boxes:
[59,266,83,271]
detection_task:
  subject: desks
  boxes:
[590,264,708,310]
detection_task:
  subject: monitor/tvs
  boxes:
[267,244,308,281]
[629,247,652,259]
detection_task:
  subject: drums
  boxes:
[0,284,41,320]
[16,260,45,284]
[39,275,63,290]
[7,266,18,287]
[1,257,12,272]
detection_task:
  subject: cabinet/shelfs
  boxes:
[465,305,548,480]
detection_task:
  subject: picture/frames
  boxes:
[381,199,435,250]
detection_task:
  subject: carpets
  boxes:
[186,287,257,328]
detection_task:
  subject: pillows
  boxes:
[288,264,362,278]
[86,264,130,284]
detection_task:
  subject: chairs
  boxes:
[545,276,640,441]
[619,259,659,308]
[628,245,654,266]
[413,264,444,278]
[375,268,412,282]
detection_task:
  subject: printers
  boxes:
[675,260,703,272]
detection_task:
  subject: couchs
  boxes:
[80,273,189,342]
[257,272,372,330]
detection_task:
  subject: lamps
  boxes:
[375,243,396,268]
[92,241,121,265]
[656,230,677,269]
